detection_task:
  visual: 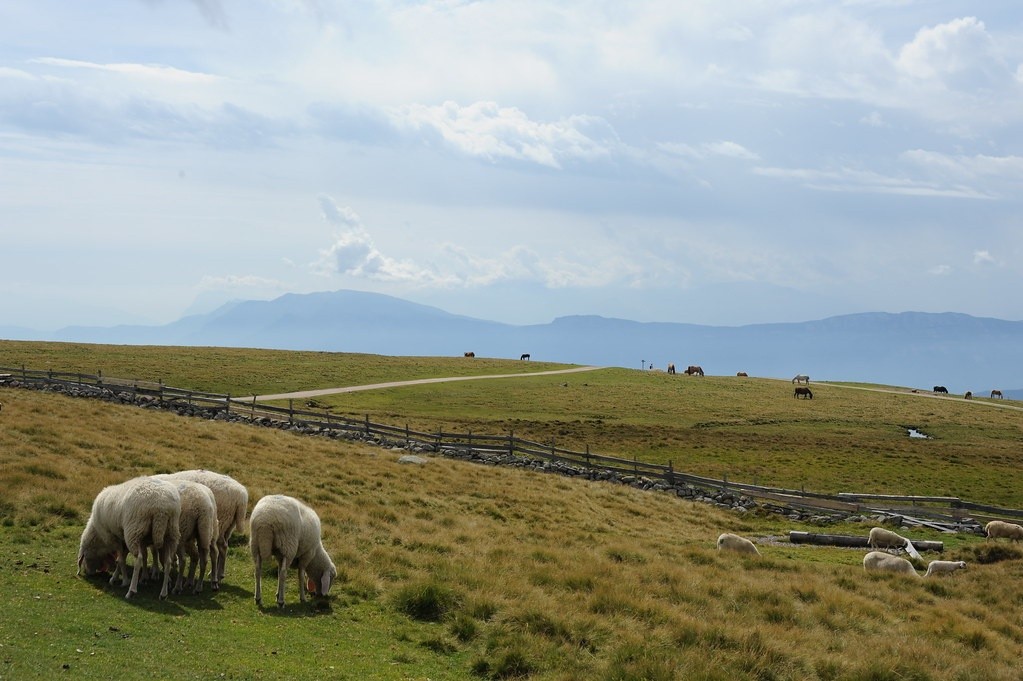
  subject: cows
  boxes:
[464,352,475,357]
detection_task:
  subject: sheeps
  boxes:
[736,371,748,377]
[248,494,336,610]
[984,520,1023,544]
[77,477,181,601]
[924,560,967,578]
[717,532,757,554]
[792,375,810,385]
[101,468,245,597]
[868,528,908,553]
[964,391,973,400]
[794,387,813,399]
[863,552,922,578]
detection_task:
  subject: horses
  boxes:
[667,363,675,374]
[520,353,530,361]
[933,385,948,394]
[990,390,1004,400]
[684,365,705,377]
